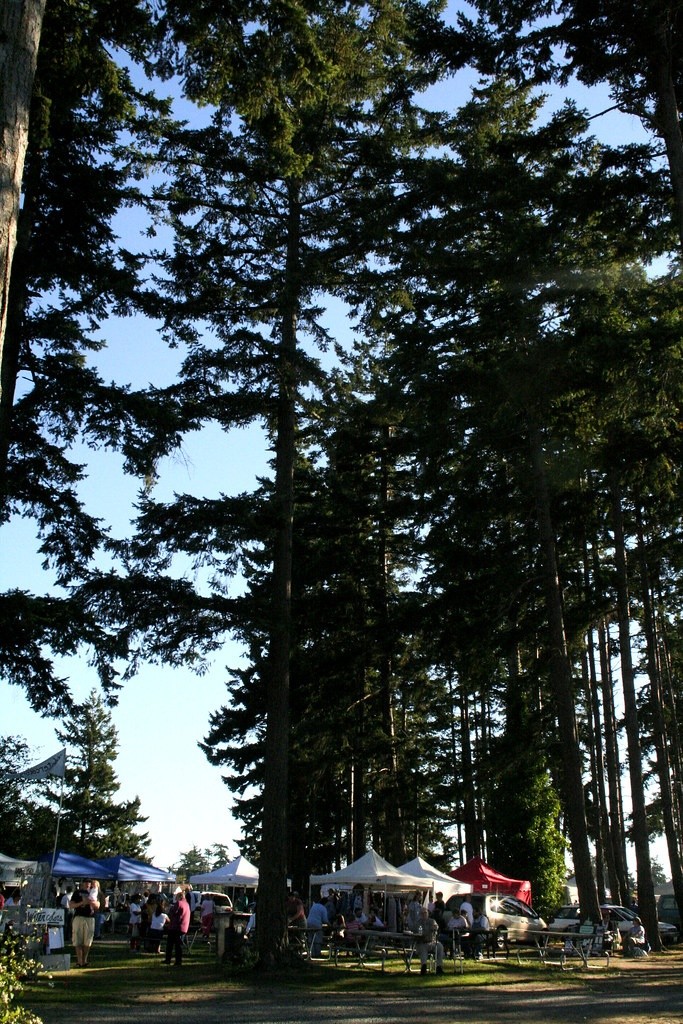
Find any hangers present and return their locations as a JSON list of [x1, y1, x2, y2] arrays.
[[389, 893, 410, 899]]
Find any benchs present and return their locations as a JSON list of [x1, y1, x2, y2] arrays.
[[376, 945, 435, 956], [548, 947, 605, 954], [514, 945, 574, 956], [306, 940, 334, 947], [333, 946, 401, 959]]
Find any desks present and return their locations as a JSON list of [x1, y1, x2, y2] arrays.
[[158, 925, 211, 956], [334, 929, 432, 974], [517, 930, 611, 970], [458, 928, 510, 957], [288, 927, 333, 962]]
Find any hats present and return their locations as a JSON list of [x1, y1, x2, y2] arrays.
[[66, 885, 73, 890], [11, 889, 22, 898], [634, 917, 643, 926], [83, 878, 92, 883]]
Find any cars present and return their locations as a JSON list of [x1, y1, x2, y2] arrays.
[[191, 891, 233, 928], [548, 903, 678, 944], [447, 892, 549, 950]]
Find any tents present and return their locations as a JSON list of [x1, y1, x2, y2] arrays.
[[0, 850, 534, 935]]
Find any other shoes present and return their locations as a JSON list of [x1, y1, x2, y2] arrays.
[[421, 969, 428, 975], [475, 954, 483, 960], [436, 966, 445, 974], [130, 948, 139, 952], [83, 963, 90, 967], [173, 963, 181, 967], [161, 959, 170, 964], [76, 964, 82, 967]]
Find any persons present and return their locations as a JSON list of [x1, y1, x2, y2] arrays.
[[0, 878, 645, 977]]
